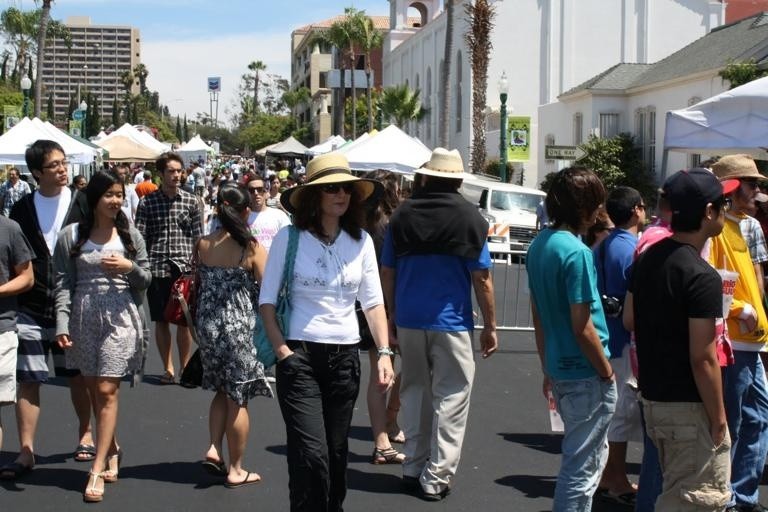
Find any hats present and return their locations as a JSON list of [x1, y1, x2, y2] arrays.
[[709, 153, 768, 186], [660, 166, 741, 221], [277, 156, 385, 218], [753, 183, 768, 204], [414, 146, 467, 180]]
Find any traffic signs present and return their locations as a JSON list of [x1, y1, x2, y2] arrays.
[[545, 147, 587, 161]]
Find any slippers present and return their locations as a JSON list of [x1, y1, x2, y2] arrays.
[[1, 460, 33, 479], [601, 489, 641, 507], [73, 443, 97, 463], [160, 373, 175, 385], [201, 458, 263, 490]]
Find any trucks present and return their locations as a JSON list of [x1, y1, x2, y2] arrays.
[[460, 174, 550, 257]]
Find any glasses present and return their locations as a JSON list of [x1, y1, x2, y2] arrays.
[[318, 181, 354, 195], [715, 197, 734, 212], [742, 177, 759, 190], [630, 203, 648, 210], [247, 186, 264, 194]]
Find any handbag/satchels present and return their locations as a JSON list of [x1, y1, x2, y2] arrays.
[[160, 274, 197, 329], [255, 291, 288, 367]]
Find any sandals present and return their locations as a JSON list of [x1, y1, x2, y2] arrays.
[[83, 445, 122, 503], [370, 445, 408, 465], [384, 422, 409, 445]]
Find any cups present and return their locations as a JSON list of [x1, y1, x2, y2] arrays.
[[711, 268, 739, 320], [547, 392, 564, 431]]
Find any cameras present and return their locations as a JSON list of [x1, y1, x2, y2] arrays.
[[600, 295, 624, 319]]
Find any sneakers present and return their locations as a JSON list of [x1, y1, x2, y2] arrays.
[[421, 473, 457, 502]]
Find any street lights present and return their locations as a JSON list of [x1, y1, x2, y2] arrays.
[[21, 74, 32, 120], [160, 97, 185, 122], [80, 100, 88, 139], [498, 72, 509, 183]]
